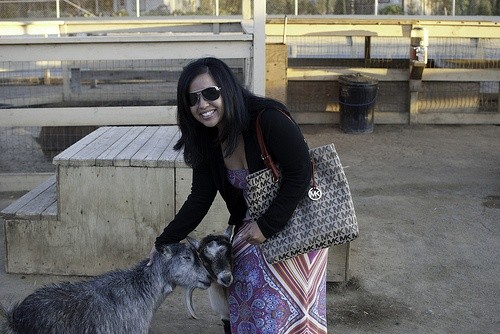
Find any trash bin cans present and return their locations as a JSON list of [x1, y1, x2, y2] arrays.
[[338, 73, 377, 134]]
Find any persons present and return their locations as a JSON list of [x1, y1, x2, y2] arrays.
[[146, 58, 329, 334]]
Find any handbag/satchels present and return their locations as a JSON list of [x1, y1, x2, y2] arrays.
[[244, 106, 360, 264]]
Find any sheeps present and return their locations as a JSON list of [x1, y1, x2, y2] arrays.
[[17, 225, 236, 334]]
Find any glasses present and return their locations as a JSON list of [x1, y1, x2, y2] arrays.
[[183, 86, 221, 107]]
